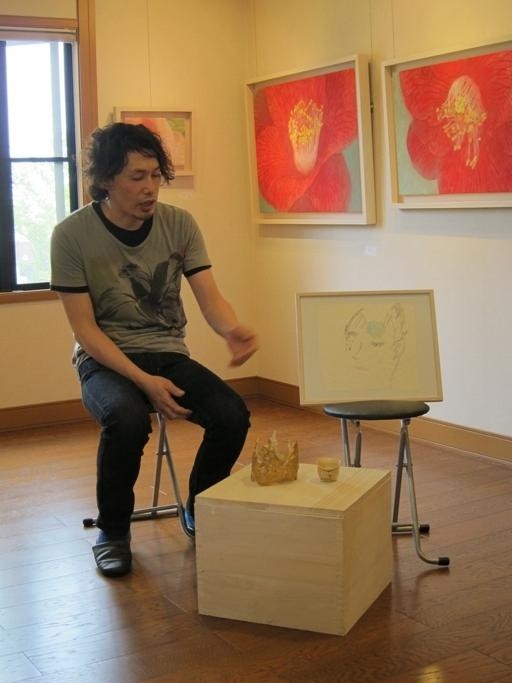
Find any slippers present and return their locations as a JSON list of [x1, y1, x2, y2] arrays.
[[92, 538, 131, 578]]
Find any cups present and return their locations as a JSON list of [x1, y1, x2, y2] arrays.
[[318, 456, 341, 481]]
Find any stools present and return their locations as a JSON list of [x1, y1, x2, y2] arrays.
[[194, 460, 393, 637], [323, 401, 451, 565], [82, 397, 195, 540]]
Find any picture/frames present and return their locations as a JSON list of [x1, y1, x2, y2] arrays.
[[112, 105, 194, 177], [295, 288, 444, 406], [380, 37, 512, 209], [244, 55, 376, 225]]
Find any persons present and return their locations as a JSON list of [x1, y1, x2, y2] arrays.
[[49, 122, 262, 577]]
